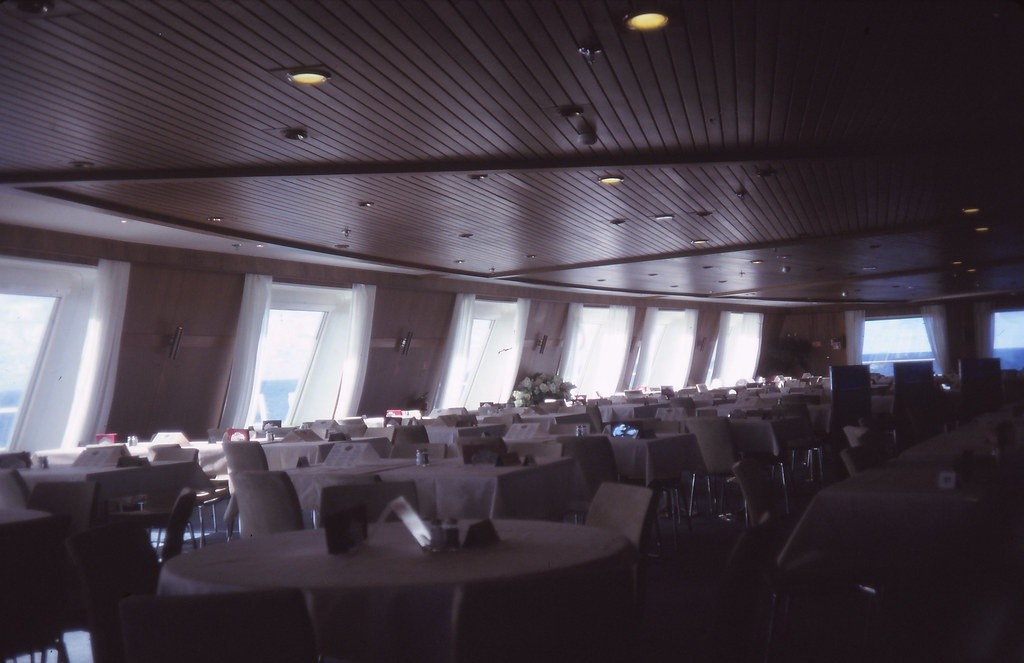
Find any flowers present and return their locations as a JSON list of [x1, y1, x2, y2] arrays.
[[510, 373, 578, 408]]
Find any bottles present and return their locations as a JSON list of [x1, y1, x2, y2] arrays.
[[416, 448, 430, 468], [127, 436, 137, 446], [576, 425, 587, 437], [37, 456, 47, 468], [429, 516, 460, 552]]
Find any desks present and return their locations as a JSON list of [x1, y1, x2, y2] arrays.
[[11, 460, 216, 526], [766, 399, 1023, 663], [655, 417, 811, 518], [598, 403, 669, 424], [279, 458, 580, 519], [364, 422, 505, 447], [477, 413, 598, 435], [37, 441, 228, 479], [158, 519, 636, 663], [254, 437, 389, 472], [502, 434, 707, 555]]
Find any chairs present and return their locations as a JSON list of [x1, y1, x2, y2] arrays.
[[0, 357, 1024, 663]]
